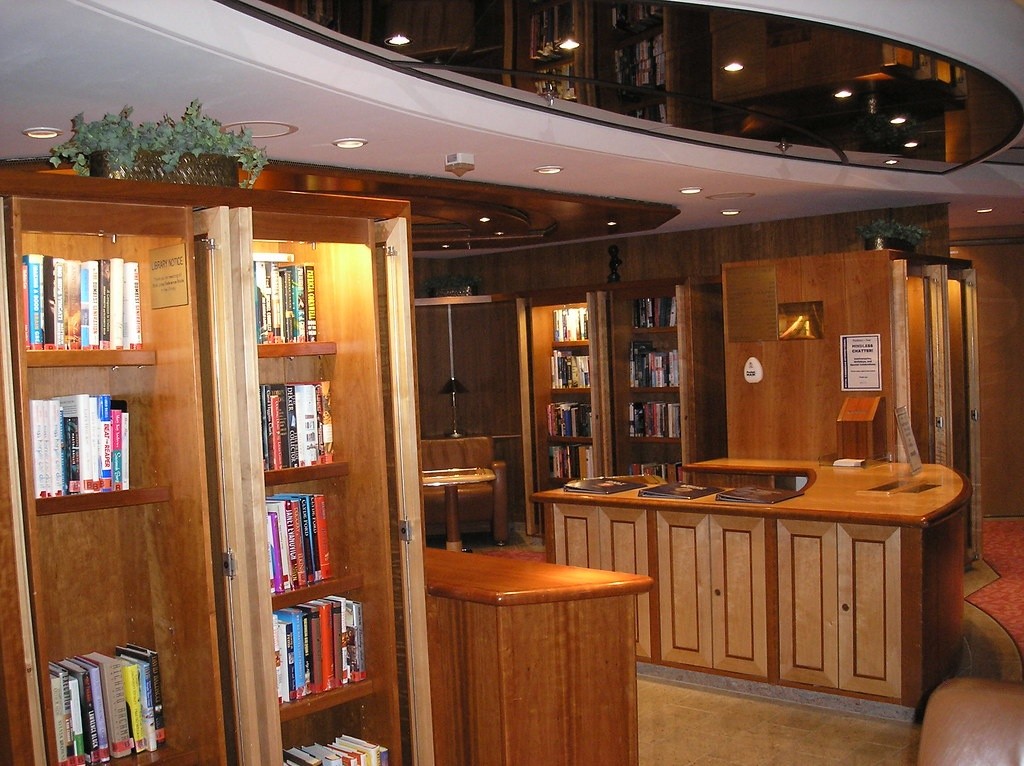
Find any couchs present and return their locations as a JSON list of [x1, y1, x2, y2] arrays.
[[421, 435, 514, 547]]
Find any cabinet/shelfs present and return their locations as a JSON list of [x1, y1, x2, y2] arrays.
[[515, 0, 596, 107], [528, 283, 713, 539], [594, 0, 715, 133], [0, 167, 441, 766]]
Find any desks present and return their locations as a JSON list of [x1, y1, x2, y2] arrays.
[[422, 469, 497, 551], [424, 546, 655, 766]]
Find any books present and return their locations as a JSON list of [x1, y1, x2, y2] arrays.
[[252, 261, 365, 701], [27, 395, 132, 501], [19, 256, 142, 351], [286, 735, 386, 766], [47, 640, 165, 766], [529, 2, 667, 123], [546, 306, 595, 479], [626, 296, 682, 484]]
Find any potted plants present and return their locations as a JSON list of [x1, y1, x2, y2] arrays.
[[49, 95, 271, 188], [855, 217, 932, 252], [416, 259, 489, 298]]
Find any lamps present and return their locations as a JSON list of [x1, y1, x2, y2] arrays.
[[438, 378, 469, 438]]
